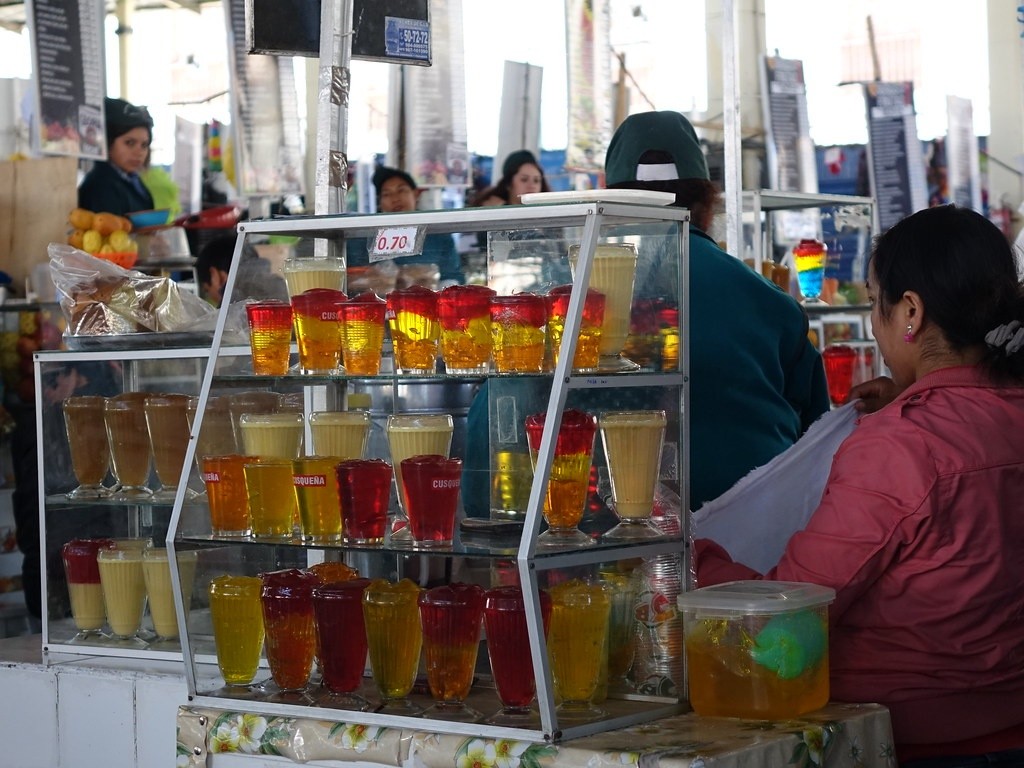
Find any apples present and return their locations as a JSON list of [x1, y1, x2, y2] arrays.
[[16, 310, 69, 375]]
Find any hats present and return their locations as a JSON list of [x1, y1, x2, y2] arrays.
[[105, 96, 150, 145], [604, 110, 710, 186]]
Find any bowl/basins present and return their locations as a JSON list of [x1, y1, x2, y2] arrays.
[[127, 210, 172, 225]]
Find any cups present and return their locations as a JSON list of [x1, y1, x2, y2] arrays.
[[822, 353, 855, 407], [740, 246, 830, 306], [489, 298, 549, 374], [384, 292, 440, 376], [439, 290, 496, 373], [546, 294, 605, 371], [286, 253, 348, 374], [291, 300, 343, 377], [64, 385, 700, 724], [569, 243, 643, 373], [247, 303, 298, 377], [333, 301, 392, 378]]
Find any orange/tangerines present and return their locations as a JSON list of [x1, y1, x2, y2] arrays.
[[68, 208, 132, 250]]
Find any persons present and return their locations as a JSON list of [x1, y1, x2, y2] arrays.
[[691, 200, 1024, 768], [341, 150, 575, 298], [463, 110, 831, 537], [13, 99, 295, 639]]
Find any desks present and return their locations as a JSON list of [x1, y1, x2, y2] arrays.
[[177, 669, 894, 768]]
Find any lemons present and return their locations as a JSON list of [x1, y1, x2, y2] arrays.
[[83, 230, 138, 254], [0, 310, 38, 370]]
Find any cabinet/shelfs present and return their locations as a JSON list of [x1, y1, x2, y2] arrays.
[[34, 185, 874, 743]]
